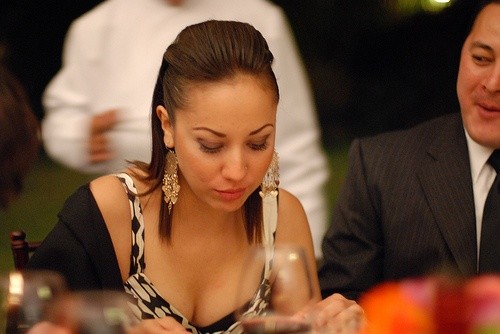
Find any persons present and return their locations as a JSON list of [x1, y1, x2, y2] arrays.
[[318, 0, 499, 306], [39, 1, 332, 262], [24, 18, 368, 334]]
